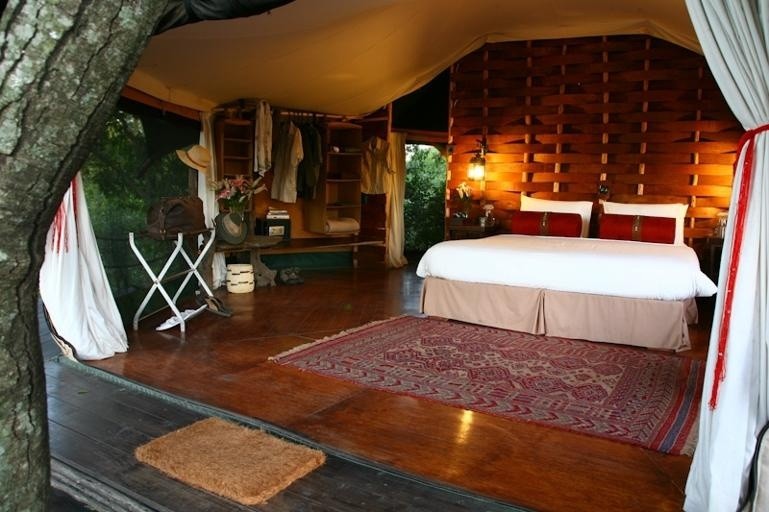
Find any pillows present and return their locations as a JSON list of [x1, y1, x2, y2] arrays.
[[511, 193, 689, 246]]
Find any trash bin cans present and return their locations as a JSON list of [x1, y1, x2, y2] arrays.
[[227, 264, 254, 293]]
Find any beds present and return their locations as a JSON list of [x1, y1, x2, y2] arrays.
[[420, 191, 692, 353]]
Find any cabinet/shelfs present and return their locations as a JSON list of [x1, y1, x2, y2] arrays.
[[214, 108, 390, 278]]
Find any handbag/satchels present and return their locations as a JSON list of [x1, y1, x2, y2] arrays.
[[148, 196, 206, 232]]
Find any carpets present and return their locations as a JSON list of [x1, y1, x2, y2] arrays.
[[133, 417, 327, 507], [270, 314, 708, 459]]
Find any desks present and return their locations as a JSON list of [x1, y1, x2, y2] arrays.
[[128, 228, 217, 336]]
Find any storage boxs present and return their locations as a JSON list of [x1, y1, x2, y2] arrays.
[[257, 217, 291, 243]]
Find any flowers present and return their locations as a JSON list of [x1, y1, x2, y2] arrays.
[[207, 174, 268, 212]]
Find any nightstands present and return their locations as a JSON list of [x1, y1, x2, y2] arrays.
[[700, 237, 725, 326], [447, 224, 498, 240]]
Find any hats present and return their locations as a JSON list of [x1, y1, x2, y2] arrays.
[[216, 212, 248, 244], [176, 145, 211, 171]]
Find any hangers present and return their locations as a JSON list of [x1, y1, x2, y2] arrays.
[[286, 110, 317, 127]]
